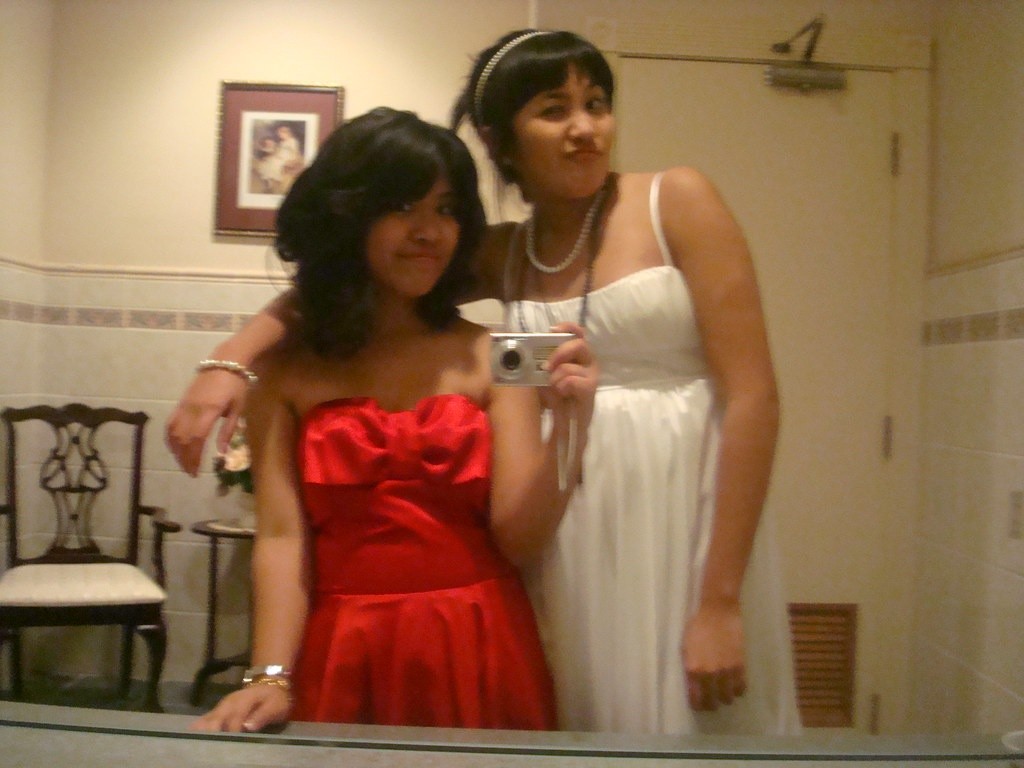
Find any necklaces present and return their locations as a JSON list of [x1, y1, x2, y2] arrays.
[[527, 197, 598, 273]]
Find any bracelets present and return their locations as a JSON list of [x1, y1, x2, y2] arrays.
[[244, 664, 293, 690], [195, 360, 258, 387]]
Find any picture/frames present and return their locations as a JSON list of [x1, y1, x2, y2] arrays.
[[214, 80, 345, 238]]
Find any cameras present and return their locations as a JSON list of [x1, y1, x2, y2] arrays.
[[488, 332, 578, 387]]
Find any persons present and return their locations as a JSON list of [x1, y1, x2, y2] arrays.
[[188, 105, 601, 734], [256, 125, 298, 194], [167, 28, 800, 737]]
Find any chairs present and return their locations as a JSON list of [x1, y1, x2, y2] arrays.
[[0, 400, 183, 712]]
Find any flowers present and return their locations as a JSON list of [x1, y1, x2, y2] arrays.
[[209, 416, 253, 497]]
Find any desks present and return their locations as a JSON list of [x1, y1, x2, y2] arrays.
[[189, 518, 255, 705]]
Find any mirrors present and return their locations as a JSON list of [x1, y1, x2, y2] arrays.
[[0, 0, 1024, 760]]
